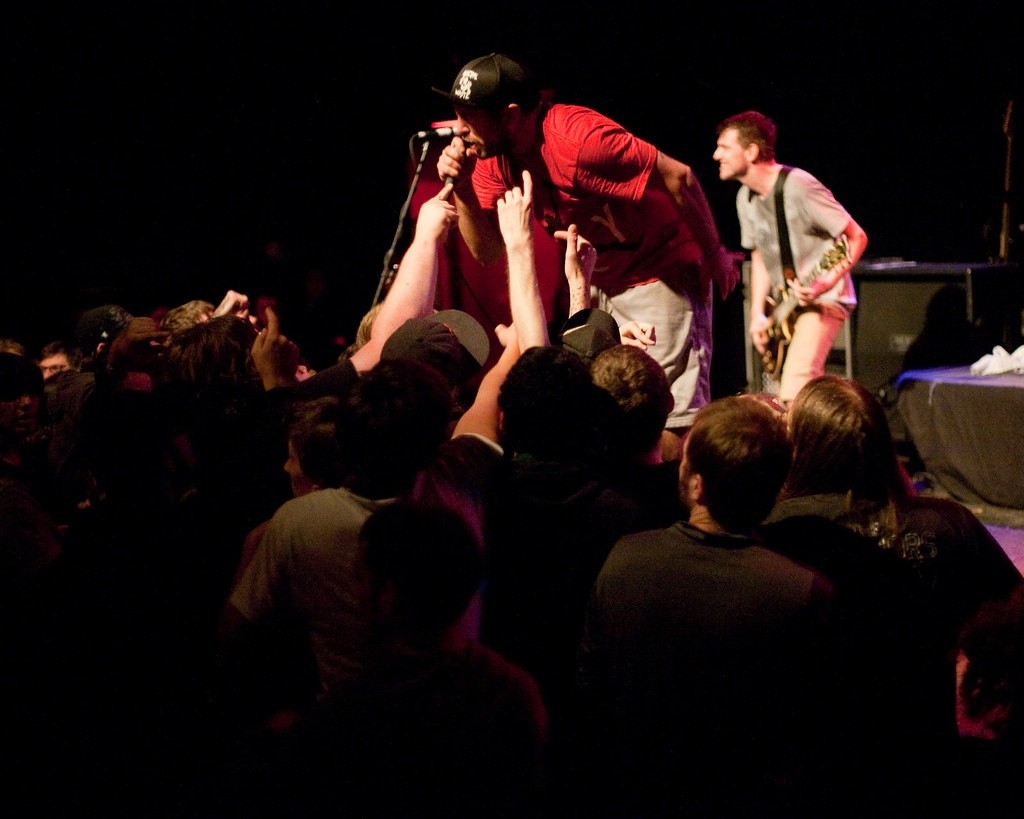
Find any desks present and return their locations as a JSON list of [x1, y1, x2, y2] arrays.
[[894, 364, 1024, 529]]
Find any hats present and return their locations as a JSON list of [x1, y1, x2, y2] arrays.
[[381, 308, 490, 415], [431, 52, 540, 111], [558, 308, 623, 366]]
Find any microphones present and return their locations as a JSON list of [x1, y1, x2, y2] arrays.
[[444, 140, 472, 201], [417, 123, 460, 140]]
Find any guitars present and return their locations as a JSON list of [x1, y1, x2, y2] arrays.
[[755, 233, 850, 380]]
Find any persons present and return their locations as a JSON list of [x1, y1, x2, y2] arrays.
[[0, 51, 1024, 818]]
